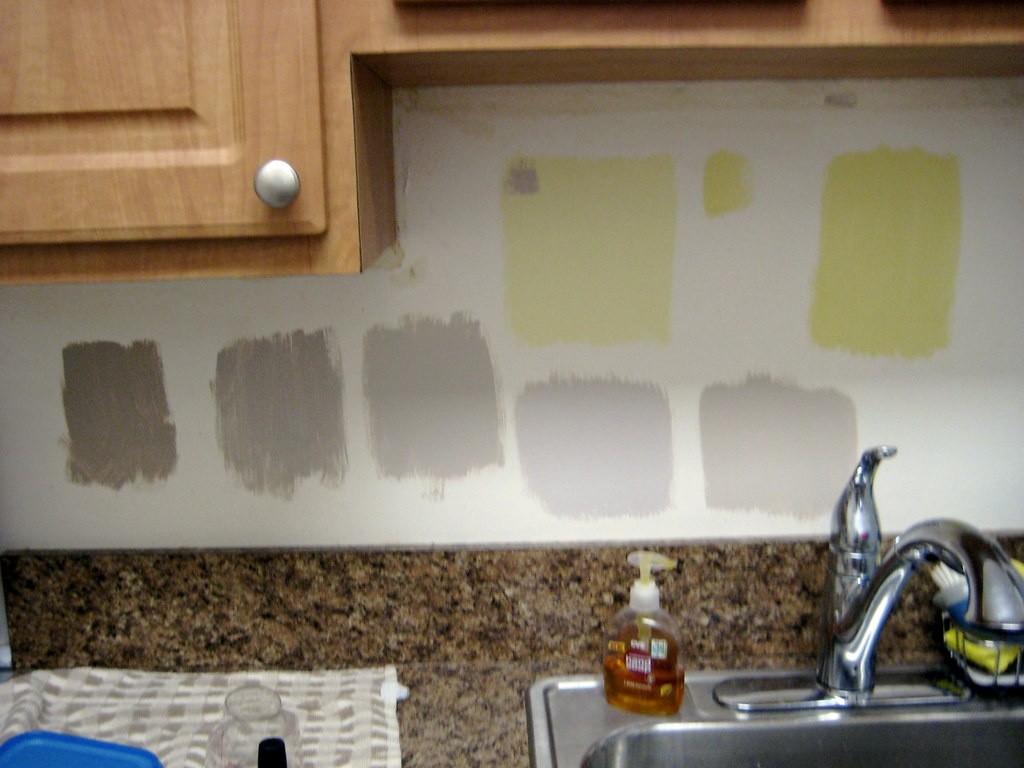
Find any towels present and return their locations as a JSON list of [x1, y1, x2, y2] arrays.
[[2, 665, 410, 766]]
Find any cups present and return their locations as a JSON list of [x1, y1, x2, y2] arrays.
[[221, 686, 304, 768]]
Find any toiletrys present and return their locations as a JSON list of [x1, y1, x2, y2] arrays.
[[603, 548, 686, 717]]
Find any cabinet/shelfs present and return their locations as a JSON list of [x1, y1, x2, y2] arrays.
[[0, 0, 1024, 283]]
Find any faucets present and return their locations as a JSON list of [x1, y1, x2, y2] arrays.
[[812, 445, 1023, 693]]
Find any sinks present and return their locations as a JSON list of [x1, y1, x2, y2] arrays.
[[527, 665, 1022, 766]]
